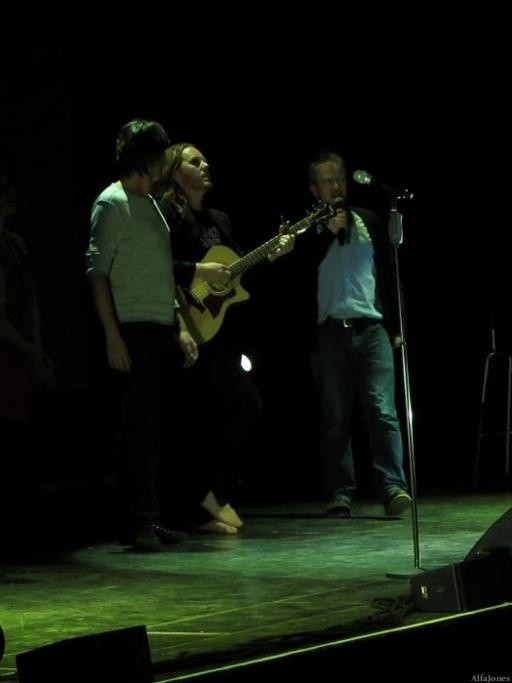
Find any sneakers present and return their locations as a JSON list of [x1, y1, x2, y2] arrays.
[[130, 510, 188, 553], [325, 498, 354, 518], [196, 490, 245, 535], [383, 486, 413, 516]]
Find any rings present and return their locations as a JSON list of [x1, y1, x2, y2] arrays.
[[332, 217, 335, 222]]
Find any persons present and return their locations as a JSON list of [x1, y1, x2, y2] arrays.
[[291, 150, 413, 518], [155, 141, 296, 536], [86, 119, 200, 548]]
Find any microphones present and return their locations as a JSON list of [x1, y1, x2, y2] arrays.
[[353, 170, 414, 202], [333, 196, 350, 246]]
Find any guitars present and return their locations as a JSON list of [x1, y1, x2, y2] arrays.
[[182, 199, 346, 345]]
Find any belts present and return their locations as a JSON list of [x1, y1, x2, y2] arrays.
[[328, 317, 362, 330]]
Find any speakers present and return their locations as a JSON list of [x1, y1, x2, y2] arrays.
[[461, 507, 512, 610], [15, 624, 156, 682]]
